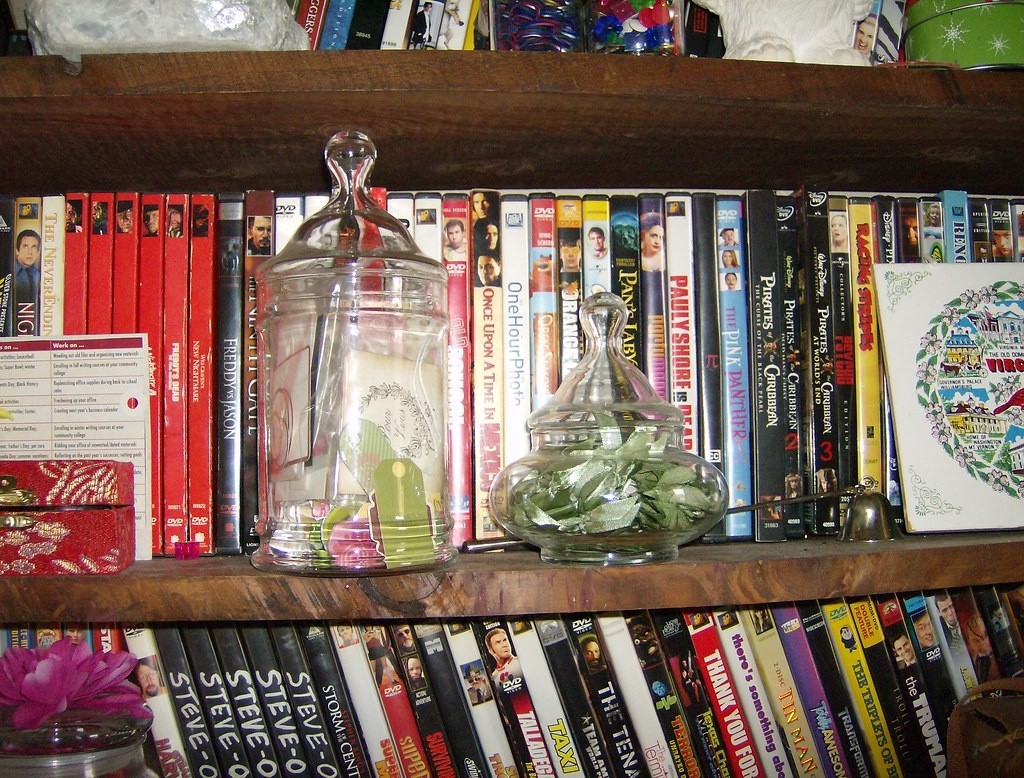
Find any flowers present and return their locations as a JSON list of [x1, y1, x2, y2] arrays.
[[0, 636, 156, 729]]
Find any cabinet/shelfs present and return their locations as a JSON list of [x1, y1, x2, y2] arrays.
[[0, 0, 1024, 778]]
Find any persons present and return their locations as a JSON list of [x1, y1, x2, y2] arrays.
[[463, 664, 492, 706], [588, 227, 606, 258], [640, 213, 664, 266], [65, 622, 86, 646], [912, 611, 937, 650], [485, 628, 524, 695], [443, 218, 468, 264], [5, 229, 41, 336], [934, 589, 966, 656], [336, 621, 360, 648], [134, 658, 166, 701], [893, 632, 917, 671], [393, 653, 427, 691], [248, 216, 271, 256], [579, 636, 607, 677], [393, 624, 417, 652]]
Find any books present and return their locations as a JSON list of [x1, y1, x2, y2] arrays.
[[0, 586, 1024, 778], [283, 0, 495, 50], [0, 186, 1024, 560], [684, 0, 726, 58]]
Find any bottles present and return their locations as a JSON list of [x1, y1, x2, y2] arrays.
[[251, 131, 459, 575], [489, 292, 730, 567]]
